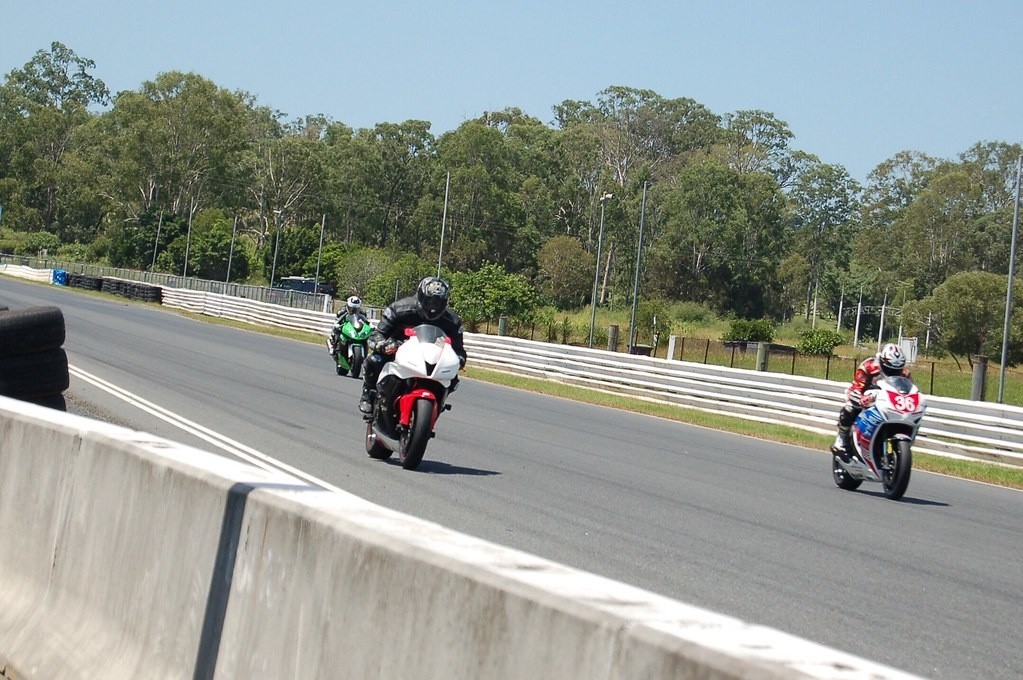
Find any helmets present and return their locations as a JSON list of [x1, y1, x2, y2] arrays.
[[417, 277, 448, 321], [878, 343, 906, 380], [346, 296, 360, 315]]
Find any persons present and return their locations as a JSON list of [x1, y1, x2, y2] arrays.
[[330, 296, 369, 358], [358, 276, 468, 413], [834, 345, 913, 458]]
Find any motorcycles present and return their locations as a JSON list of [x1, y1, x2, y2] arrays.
[[359, 323, 464, 469], [326, 312, 370, 377], [830, 374, 927, 499]]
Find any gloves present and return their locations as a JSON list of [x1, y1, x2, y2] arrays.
[[379, 337, 397, 354], [860, 394, 876, 409]]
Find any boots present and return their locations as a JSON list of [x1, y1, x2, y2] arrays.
[[833, 423, 851, 455], [358, 355, 382, 414]]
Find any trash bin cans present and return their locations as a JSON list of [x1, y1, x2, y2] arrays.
[[608, 325, 619, 351], [755, 342, 769, 371], [499, 316, 507, 336], [627, 344, 654, 356]]
